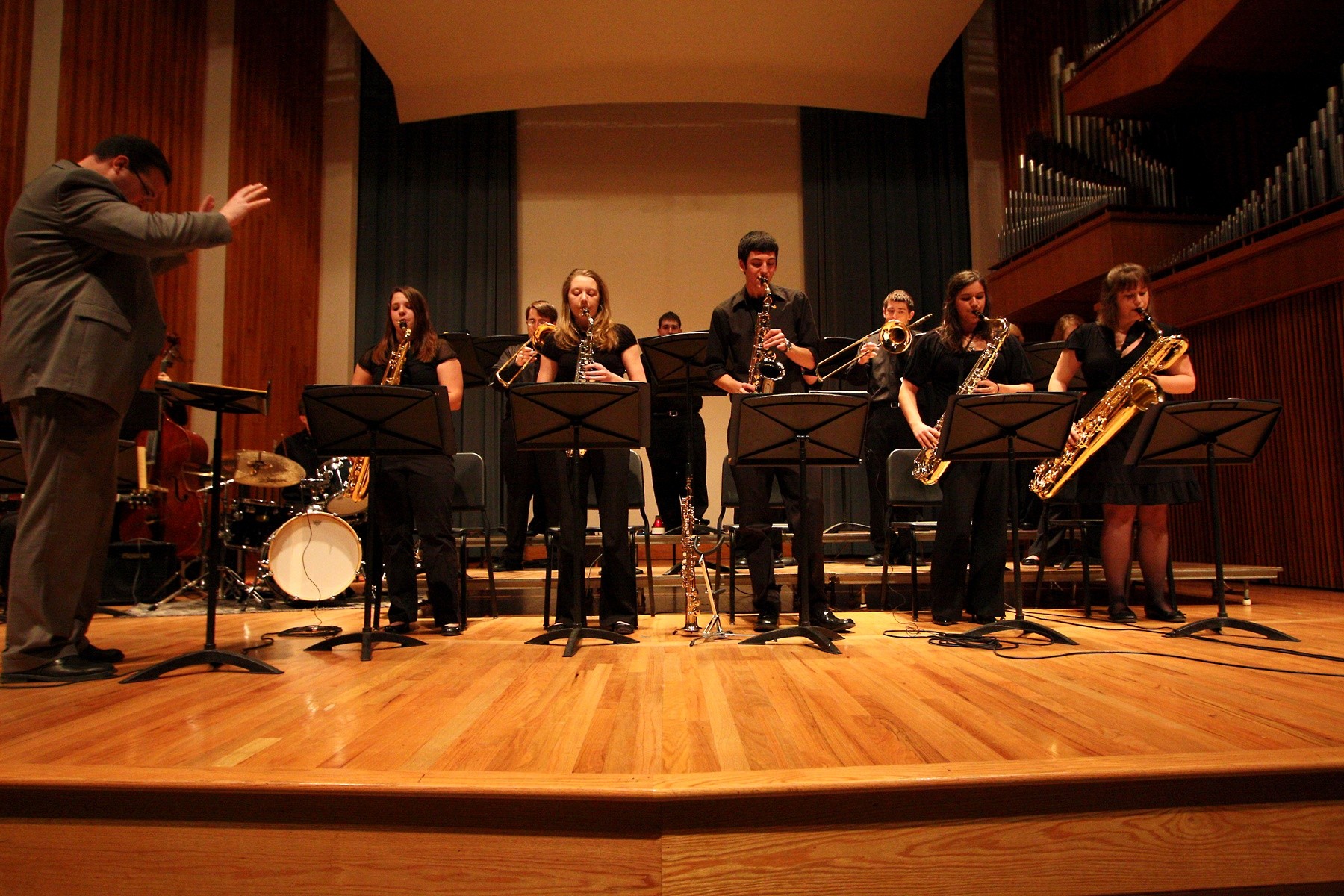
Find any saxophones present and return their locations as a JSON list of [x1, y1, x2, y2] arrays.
[[910, 310, 1012, 486], [1027, 306, 1190, 501], [749, 275, 785, 407], [679, 462, 704, 632], [564, 309, 598, 458], [345, 318, 413, 501]]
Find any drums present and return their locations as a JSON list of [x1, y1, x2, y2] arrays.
[[316, 455, 370, 518], [223, 496, 300, 551], [298, 476, 328, 514], [257, 505, 364, 605]]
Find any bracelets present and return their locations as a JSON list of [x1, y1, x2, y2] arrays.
[[784, 336, 792, 353]]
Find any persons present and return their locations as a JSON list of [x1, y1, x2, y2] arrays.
[[491, 299, 559, 571], [904, 276, 1034, 626], [708, 231, 856, 632], [645, 310, 711, 536], [845, 290, 926, 566], [1048, 263, 1196, 623], [351, 285, 464, 635], [1, 133, 271, 679], [1000, 312, 1084, 566], [538, 269, 647, 634]]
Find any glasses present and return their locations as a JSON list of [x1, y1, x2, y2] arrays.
[[526, 320, 550, 327], [128, 163, 157, 199]]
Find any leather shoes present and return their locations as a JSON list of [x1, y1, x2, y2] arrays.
[[61, 643, 125, 668], [972, 611, 996, 626], [865, 553, 885, 565], [800, 608, 855, 632], [934, 613, 953, 626], [1143, 598, 1186, 621], [897, 551, 926, 566], [1108, 602, 1136, 621], [754, 609, 780, 630], [0, 658, 117, 683], [735, 556, 751, 568], [773, 558, 783, 567]]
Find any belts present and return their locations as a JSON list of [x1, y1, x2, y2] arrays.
[[656, 410, 685, 417]]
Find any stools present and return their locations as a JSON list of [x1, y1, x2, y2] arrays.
[[102, 540, 177, 609]]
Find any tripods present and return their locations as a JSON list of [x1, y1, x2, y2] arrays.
[[1121, 398, 1303, 642], [935, 340, 1106, 645], [119, 381, 284, 684], [302, 384, 456, 662], [508, 381, 651, 658], [640, 328, 874, 650]]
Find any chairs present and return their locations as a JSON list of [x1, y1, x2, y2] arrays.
[[878, 448, 944, 622], [713, 455, 794, 628], [413, 452, 498, 632], [543, 450, 655, 629], [1035, 479, 1177, 617]]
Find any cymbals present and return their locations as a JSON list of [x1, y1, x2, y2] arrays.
[[209, 450, 307, 489], [183, 469, 227, 480]]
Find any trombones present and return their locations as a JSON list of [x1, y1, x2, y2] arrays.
[[815, 311, 935, 383], [496, 323, 560, 389]]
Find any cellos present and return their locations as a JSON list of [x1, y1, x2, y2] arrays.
[[118, 330, 209, 559]]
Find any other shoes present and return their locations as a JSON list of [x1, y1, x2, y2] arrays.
[[616, 620, 635, 633], [492, 562, 523, 572], [1021, 556, 1040, 565], [441, 620, 462, 635], [548, 619, 587, 631], [384, 621, 410, 633]]
